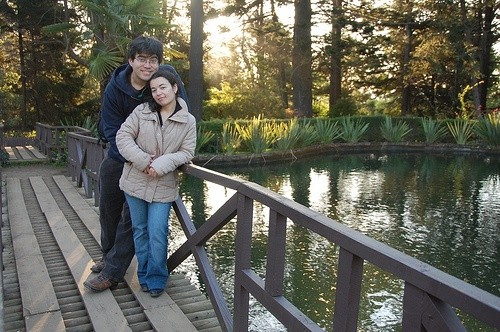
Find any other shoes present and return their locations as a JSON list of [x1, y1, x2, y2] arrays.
[[85, 272, 118, 292], [90, 261, 107, 272]]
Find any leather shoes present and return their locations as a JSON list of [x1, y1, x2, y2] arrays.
[[141, 283, 149, 291], [149, 288, 164, 296]]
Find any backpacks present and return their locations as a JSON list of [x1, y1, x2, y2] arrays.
[[95, 72, 114, 146]]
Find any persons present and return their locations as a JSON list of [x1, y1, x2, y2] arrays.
[[83, 36, 190, 292], [116, 70, 196, 298]]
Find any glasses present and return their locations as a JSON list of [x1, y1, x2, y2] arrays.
[[134, 57, 158, 65]]
